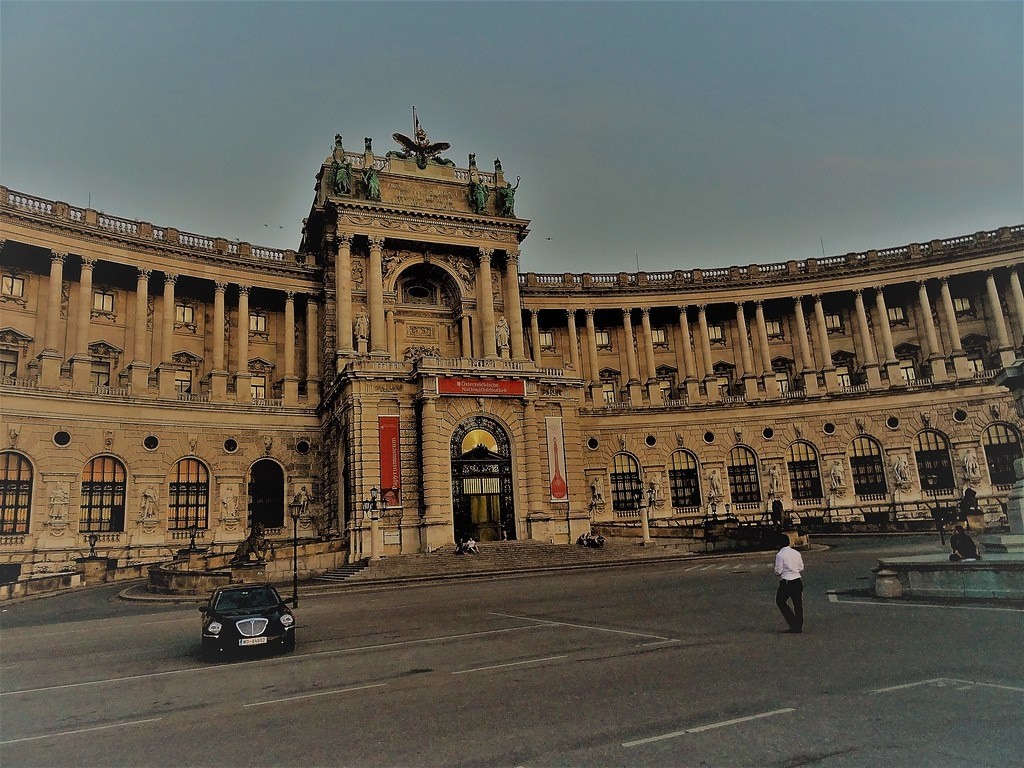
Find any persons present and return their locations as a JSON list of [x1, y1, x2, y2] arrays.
[[496, 316, 510, 348], [355, 306, 369, 337], [830, 460, 846, 490], [221, 485, 238, 518], [949, 525, 982, 561], [382, 249, 407, 281], [963, 448, 981, 478], [448, 253, 471, 279], [50, 482, 71, 521], [709, 469, 721, 496], [645, 480, 658, 519], [768, 463, 784, 492], [590, 476, 604, 501], [468, 175, 521, 214], [893, 454, 911, 481], [294, 487, 314, 514], [142, 484, 161, 518], [332, 155, 387, 198], [455, 538, 481, 555], [776, 535, 804, 631], [576, 532, 606, 548], [402, 345, 442, 363]]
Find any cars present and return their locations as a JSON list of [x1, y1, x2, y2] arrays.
[[199, 581, 297, 660]]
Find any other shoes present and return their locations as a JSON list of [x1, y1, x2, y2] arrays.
[[779, 627, 803, 633]]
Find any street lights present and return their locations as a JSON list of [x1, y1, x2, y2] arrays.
[[287, 495, 303, 612]]
